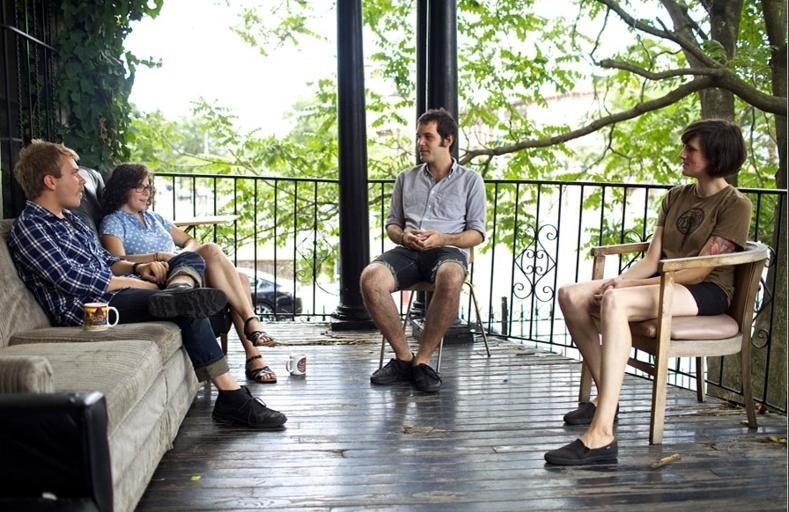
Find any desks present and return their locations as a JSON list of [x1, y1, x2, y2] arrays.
[[171, 213, 241, 243]]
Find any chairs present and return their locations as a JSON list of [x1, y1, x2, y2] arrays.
[[577, 238, 767, 445], [377, 246, 492, 376], [70, 163, 233, 355]]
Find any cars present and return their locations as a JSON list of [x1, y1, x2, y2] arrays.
[[230, 266, 306, 327], [151, 173, 216, 212]]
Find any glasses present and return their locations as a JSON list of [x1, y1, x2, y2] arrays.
[[132, 184, 153, 193]]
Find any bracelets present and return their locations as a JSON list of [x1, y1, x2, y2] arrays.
[[153, 252, 160, 263], [131, 261, 143, 277]]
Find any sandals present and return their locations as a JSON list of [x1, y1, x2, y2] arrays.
[[244, 355, 278, 383], [242, 316, 274, 346]]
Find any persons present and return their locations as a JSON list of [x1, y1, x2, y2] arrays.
[[360, 108, 486, 390], [543, 118, 752, 467], [99, 162, 276, 384], [7, 139, 288, 428]]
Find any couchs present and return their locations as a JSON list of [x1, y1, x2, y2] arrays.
[[0, 217, 208, 512]]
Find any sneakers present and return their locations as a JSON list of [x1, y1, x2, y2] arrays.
[[370, 352, 415, 386], [147, 283, 228, 320], [211, 386, 287, 428], [543, 437, 619, 465], [563, 400, 620, 425], [411, 363, 443, 393]]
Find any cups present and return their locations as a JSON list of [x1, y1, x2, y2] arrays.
[[84, 303, 119, 332], [287, 352, 307, 376]]
